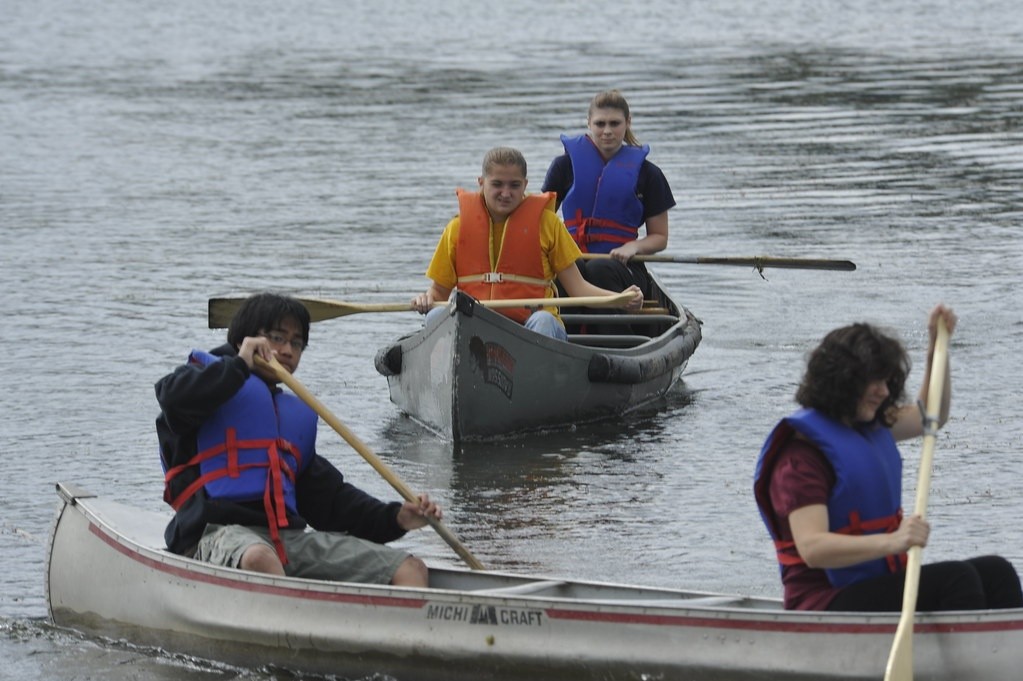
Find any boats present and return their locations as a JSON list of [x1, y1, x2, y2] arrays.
[[374, 265, 702, 445], [45, 480, 1023, 681]]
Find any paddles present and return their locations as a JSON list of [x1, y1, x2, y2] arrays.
[[255, 346, 486, 569], [580, 252, 857, 272], [879, 315, 950, 680], [208, 291, 634, 331]]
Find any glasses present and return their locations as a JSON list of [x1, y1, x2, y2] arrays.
[[258, 332, 307, 350]]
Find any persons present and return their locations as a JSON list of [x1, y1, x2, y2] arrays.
[[411, 146, 644, 342], [154, 293, 442, 588], [541, 91, 676, 348], [753, 304, 1023, 613]]
